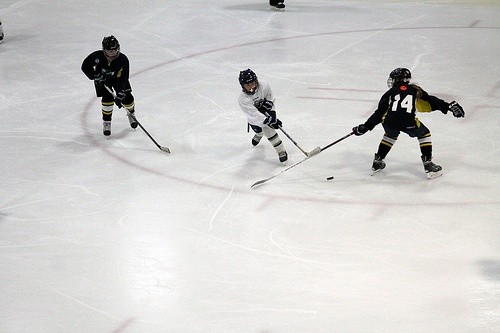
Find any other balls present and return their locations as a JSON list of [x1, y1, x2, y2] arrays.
[[327, 176, 334, 181]]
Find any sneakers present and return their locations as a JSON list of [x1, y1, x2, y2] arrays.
[[425, 163, 443, 179], [102, 118, 112, 140], [279, 151, 288, 166], [128, 112, 139, 132], [0, 22, 5, 44], [269, 1, 285, 11], [370, 162, 387, 176], [252, 134, 264, 148]]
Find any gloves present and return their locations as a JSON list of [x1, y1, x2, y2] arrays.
[[259, 100, 273, 114], [94, 73, 106, 86], [353, 124, 367, 136], [113, 92, 125, 108], [263, 117, 283, 130], [448, 101, 465, 118]]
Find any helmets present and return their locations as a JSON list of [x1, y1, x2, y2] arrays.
[[387, 69, 411, 88], [102, 36, 120, 59], [239, 69, 258, 95]]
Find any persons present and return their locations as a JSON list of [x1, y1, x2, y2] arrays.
[[0, 22, 4, 44], [238, 68, 288, 166], [353, 68, 465, 179], [81, 35, 138, 139], [269, 0, 285, 12]]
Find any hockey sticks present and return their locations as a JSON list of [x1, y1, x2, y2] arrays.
[[251, 131, 354, 188], [103, 84, 170, 154], [265, 111, 321, 158]]
